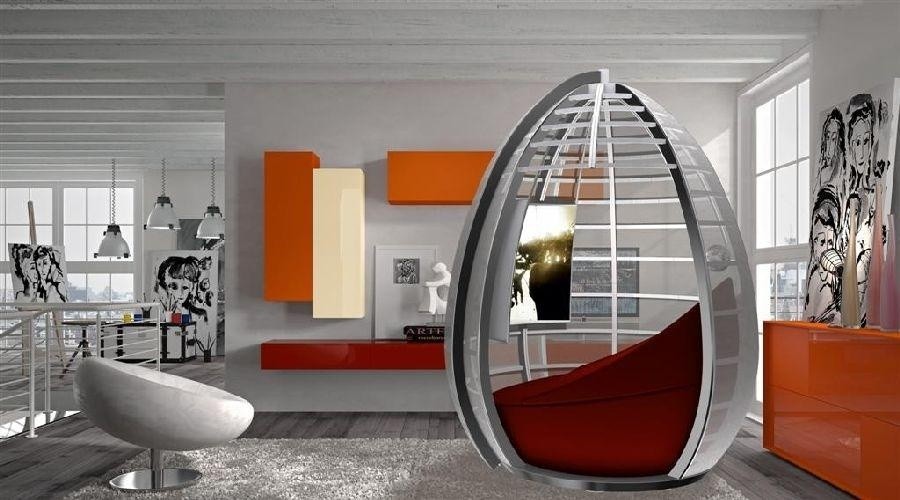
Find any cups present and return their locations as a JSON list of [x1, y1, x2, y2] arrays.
[[172, 313, 181, 324], [123, 314, 131, 323], [143, 311, 150, 318], [166, 315, 172, 323], [134, 314, 142, 322], [182, 314, 189, 324]]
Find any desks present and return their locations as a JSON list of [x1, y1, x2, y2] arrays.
[[762, 320, 900, 498], [60, 320, 197, 379]]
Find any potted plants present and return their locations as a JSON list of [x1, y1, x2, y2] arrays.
[[196, 331, 216, 362]]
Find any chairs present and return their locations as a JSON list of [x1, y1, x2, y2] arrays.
[[74, 357, 254, 493], [493, 279, 735, 477]]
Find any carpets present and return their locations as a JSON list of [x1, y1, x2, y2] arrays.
[[64, 435, 740, 498]]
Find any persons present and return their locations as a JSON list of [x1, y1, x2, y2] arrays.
[[807, 186, 844, 325], [812, 107, 845, 191], [844, 94, 889, 191], [10, 244, 44, 302], [34, 246, 66, 302], [155, 254, 208, 321]]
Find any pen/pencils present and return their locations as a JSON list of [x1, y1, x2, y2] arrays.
[[136, 293, 155, 310], [160, 292, 177, 310]]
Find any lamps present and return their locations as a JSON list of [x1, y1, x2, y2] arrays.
[[93, 157, 226, 259]]
